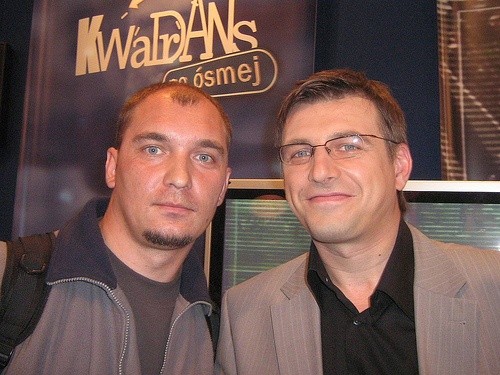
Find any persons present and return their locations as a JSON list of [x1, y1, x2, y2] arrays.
[[217, 72, 500, 375], [0, 82, 232, 375]]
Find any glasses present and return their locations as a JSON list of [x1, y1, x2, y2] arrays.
[[276, 134, 369, 166]]
